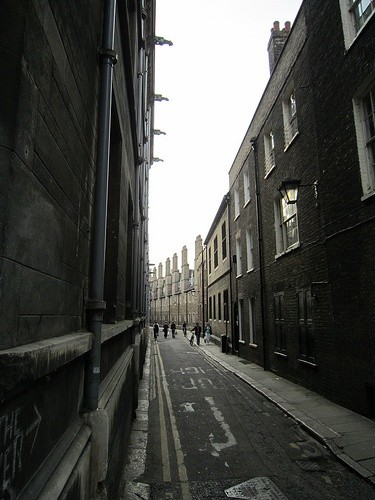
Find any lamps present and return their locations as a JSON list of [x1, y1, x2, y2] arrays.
[[276, 178, 319, 207]]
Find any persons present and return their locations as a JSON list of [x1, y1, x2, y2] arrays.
[[204, 323, 212, 344], [171, 322, 176, 338], [182, 321, 187, 336], [163, 321, 169, 338], [153, 321, 159, 338], [190, 323, 201, 346]]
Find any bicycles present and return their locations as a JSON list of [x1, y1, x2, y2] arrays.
[[188, 332, 195, 346]]
